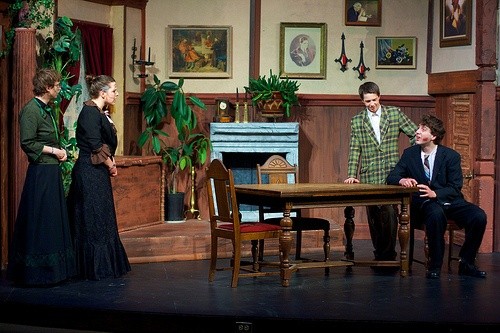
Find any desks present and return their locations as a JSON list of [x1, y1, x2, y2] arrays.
[[222, 185, 418, 287]]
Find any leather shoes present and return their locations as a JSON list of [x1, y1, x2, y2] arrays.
[[458, 261, 487, 276], [426, 270, 441, 279]]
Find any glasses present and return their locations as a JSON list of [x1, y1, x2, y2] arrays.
[[54, 84, 60, 88]]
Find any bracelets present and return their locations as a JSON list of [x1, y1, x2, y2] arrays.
[[52, 147, 54, 155]]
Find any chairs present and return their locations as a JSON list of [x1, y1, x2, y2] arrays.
[[251, 155, 333, 280], [406, 195, 466, 280], [203, 158, 285, 288]]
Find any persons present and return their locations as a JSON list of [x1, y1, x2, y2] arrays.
[[6, 68, 81, 287], [345, 82, 419, 261], [66, 75, 131, 282], [385, 114, 487, 279]]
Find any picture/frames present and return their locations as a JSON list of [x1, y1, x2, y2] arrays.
[[279, 22, 327, 80], [375, 36, 417, 69], [167, 24, 232, 78], [345, 0, 383, 27], [438, 0, 472, 47]]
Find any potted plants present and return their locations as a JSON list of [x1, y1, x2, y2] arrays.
[[245, 70, 300, 116], [135, 75, 212, 222]]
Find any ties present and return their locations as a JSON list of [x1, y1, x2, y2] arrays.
[[424, 155, 430, 184]]
